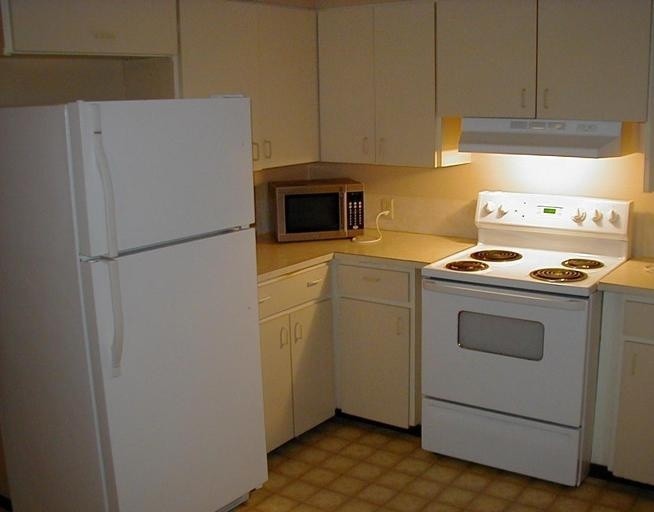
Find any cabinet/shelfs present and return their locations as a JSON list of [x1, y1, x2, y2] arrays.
[[334, 262, 413, 428], [613, 299, 654, 488], [435, 0, 649, 119], [9, 0, 174, 55], [256, 260, 335, 454], [317, 1, 435, 168], [178, 1, 320, 170]]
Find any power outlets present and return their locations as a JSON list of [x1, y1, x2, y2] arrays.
[[381, 198, 394, 220]]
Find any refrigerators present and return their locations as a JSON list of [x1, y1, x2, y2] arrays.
[[0, 98, 267, 511]]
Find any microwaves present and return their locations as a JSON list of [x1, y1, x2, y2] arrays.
[[269, 178, 366, 243]]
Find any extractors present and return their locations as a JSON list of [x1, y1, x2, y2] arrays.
[[459, 119, 644, 158]]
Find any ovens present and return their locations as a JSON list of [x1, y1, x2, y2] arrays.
[[421, 277, 603, 428]]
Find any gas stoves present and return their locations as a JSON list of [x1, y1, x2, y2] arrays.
[[422, 190, 633, 297]]
